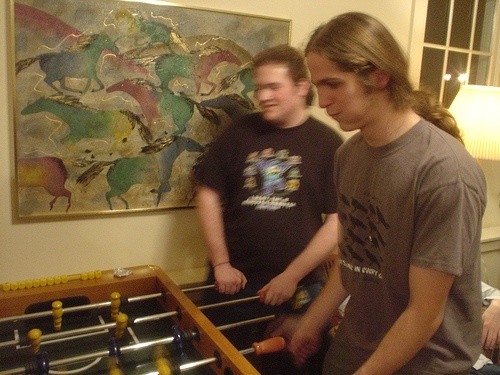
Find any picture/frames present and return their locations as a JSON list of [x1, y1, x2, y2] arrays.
[[5, 0, 295, 227]]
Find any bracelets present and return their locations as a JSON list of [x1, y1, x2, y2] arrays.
[[212, 261, 231, 267]]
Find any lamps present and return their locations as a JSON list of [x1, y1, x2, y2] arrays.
[[443, 83, 500, 163]]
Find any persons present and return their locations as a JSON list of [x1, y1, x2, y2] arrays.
[[192, 45, 344, 375], [289, 11, 488, 375]]
